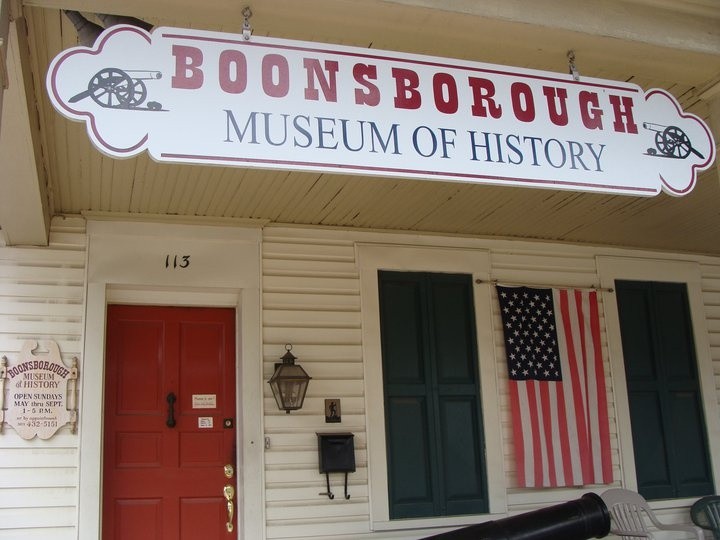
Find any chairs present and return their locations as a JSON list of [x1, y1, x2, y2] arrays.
[[690, 495, 720, 540], [599, 489, 706, 540]]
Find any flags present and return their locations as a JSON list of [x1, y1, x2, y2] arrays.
[[496, 281, 614, 488]]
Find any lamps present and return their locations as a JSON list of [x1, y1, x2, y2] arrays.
[[266, 343, 313, 414]]
